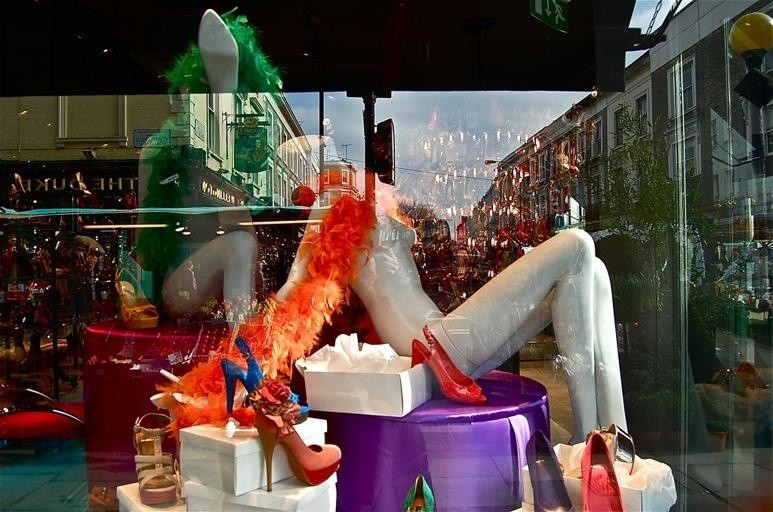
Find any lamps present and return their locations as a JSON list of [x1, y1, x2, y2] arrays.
[[82, 149, 96, 161]]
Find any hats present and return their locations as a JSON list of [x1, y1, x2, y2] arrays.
[[72, 235, 106, 257]]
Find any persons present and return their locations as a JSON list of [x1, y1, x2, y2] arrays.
[[135, 9, 282, 331], [0, 228, 107, 368], [267, 133, 630, 443], [641, 219, 729, 497]]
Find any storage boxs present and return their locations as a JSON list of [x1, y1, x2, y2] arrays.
[[179, 468, 339, 512], [302, 354, 436, 419], [178, 413, 330, 498], [114, 481, 189, 512]]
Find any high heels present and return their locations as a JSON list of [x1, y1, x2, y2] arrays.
[[221, 338, 310, 424], [254, 410, 341, 492], [581, 432, 624, 511], [113, 268, 159, 329], [132, 412, 177, 506], [411, 326, 487, 405], [527, 429, 574, 512], [403, 474, 435, 512]]
